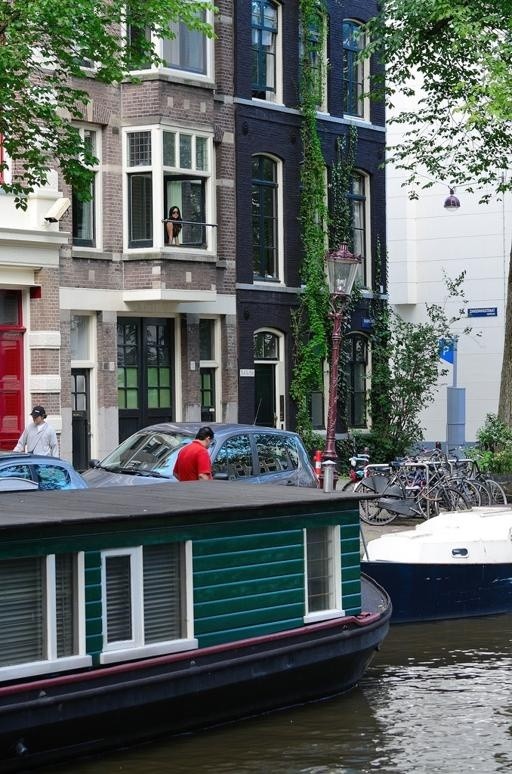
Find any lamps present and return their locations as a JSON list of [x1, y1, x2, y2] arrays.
[[442, 182, 461, 211]]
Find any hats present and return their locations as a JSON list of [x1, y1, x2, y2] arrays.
[[30, 407, 44, 416]]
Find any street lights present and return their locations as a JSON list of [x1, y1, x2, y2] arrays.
[[323, 236, 362, 495]]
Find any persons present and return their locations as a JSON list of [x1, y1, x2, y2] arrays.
[[173, 426, 214, 482], [13, 405, 60, 459], [164, 205, 183, 245]]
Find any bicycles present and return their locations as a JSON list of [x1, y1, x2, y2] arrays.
[[341, 445, 507, 526]]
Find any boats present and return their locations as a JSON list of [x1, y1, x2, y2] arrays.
[[0, 477, 393, 770], [356, 501, 512, 623]]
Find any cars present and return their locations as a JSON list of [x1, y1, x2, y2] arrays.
[[0, 448, 90, 494], [82, 422, 319, 487]]
